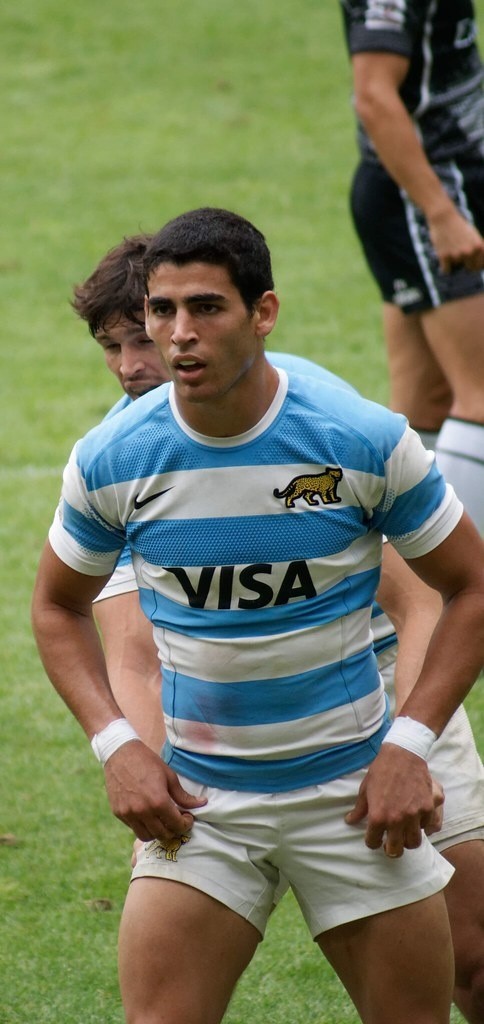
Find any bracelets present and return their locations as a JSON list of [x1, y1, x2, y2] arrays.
[[380, 716, 436, 762], [90, 718, 141, 766]]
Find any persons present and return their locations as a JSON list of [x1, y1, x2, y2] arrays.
[[339, 0, 484, 541], [32, 208, 484, 1024]]
